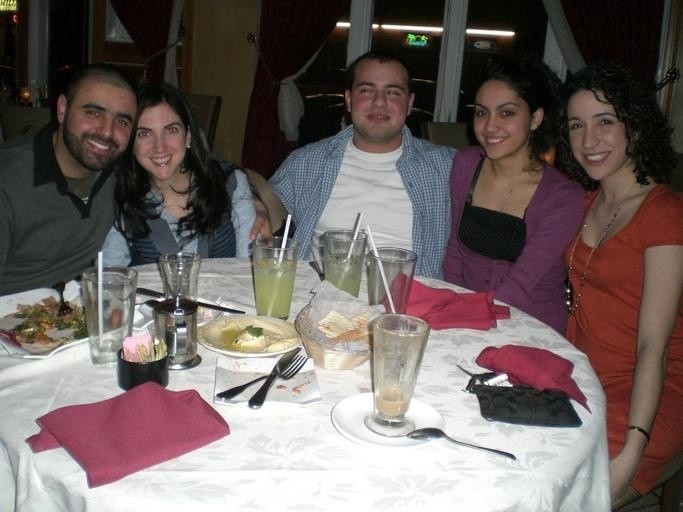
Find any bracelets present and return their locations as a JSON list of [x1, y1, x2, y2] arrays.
[[626, 423, 652, 446]]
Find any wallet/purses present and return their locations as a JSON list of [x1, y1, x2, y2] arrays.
[[473, 385, 583, 427]]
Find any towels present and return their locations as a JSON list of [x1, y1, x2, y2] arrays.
[[476, 345, 592, 414], [28, 381, 230, 487], [381, 273, 510, 330]]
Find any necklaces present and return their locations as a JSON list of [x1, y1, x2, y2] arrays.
[[564, 183, 638, 316]]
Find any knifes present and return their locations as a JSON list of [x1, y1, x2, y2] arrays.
[[247, 345, 298, 409], [128, 284, 247, 320]]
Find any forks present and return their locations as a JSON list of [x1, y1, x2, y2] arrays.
[[50, 281, 74, 318], [215, 355, 310, 401]]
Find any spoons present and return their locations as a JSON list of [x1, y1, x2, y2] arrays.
[[407, 427, 519, 464]]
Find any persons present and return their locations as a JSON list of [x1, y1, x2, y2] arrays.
[[0, 64, 273, 305], [112, 82, 264, 267], [555, 59, 682, 505], [442, 58, 589, 335], [266, 47, 459, 282]]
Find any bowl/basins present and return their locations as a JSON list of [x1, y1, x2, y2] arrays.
[[295, 304, 373, 371]]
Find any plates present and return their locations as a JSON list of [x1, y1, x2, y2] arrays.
[[141, 293, 221, 326], [331, 390, 444, 453], [193, 316, 302, 358], [1, 280, 154, 360]]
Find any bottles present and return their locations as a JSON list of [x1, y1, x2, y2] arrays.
[[153, 290, 200, 371]]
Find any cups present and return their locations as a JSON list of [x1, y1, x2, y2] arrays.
[[78, 265, 141, 367], [252, 235, 302, 319], [113, 348, 170, 392], [363, 313, 431, 440], [321, 229, 369, 299], [157, 249, 199, 307], [368, 245, 418, 315]]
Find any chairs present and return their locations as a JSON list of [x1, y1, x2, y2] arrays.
[[421, 122, 470, 146], [181, 94, 222, 150]]
